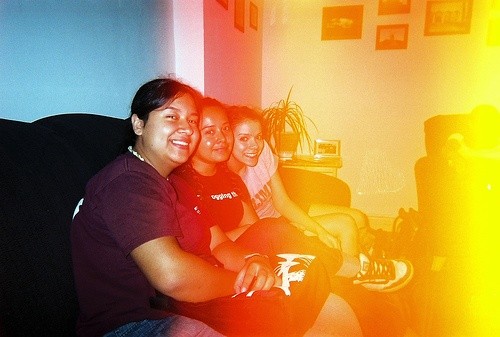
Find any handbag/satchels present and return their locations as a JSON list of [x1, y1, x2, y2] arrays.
[[182, 217, 331, 337]]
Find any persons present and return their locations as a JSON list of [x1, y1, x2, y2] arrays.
[[228, 105, 369, 258], [71, 78, 283, 337], [167, 96, 414, 337]]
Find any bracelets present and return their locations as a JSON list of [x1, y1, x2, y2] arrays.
[[243, 252, 270, 261]]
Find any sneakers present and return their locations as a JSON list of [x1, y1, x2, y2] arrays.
[[351, 254, 414, 293]]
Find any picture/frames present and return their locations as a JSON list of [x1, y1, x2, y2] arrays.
[[315, 140, 341, 157], [375, 25, 408, 49], [234, 0, 245, 33], [249, 2, 258, 30], [424, 0, 473, 35], [378, 0, 410, 15], [321, 6, 363, 40], [216, 0, 228, 10]]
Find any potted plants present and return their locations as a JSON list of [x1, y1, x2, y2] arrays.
[[262, 84, 319, 159]]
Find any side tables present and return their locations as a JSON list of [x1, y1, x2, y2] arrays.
[[279, 156, 343, 177]]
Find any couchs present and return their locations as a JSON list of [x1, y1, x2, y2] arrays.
[[0, 114, 350, 337]]
